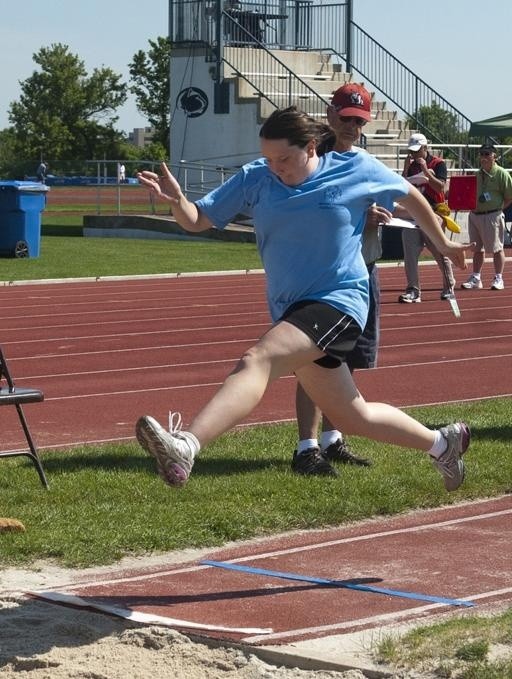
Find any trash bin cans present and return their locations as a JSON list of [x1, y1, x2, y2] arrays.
[[0, 180, 51, 260]]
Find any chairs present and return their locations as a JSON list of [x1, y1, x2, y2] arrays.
[[0, 350, 53, 493]]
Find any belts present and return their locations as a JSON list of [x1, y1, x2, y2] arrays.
[[471, 208, 503, 214]]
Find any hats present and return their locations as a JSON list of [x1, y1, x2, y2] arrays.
[[479, 144, 498, 153], [407, 133, 427, 152], [332, 83, 372, 122]]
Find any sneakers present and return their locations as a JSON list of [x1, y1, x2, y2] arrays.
[[135, 415, 194, 488], [399, 290, 422, 303], [462, 275, 484, 289], [441, 291, 456, 300], [430, 422, 470, 491], [491, 276, 504, 290], [291, 447, 341, 479], [319, 439, 372, 466]]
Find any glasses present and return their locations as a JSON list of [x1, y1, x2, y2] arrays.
[[480, 154, 491, 156], [336, 116, 366, 125]]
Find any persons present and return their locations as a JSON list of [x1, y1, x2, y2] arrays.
[[120, 163, 126, 184], [460, 142, 512, 291], [289, 82, 386, 480], [131, 104, 479, 488], [397, 133, 457, 305], [35, 162, 49, 184]]
